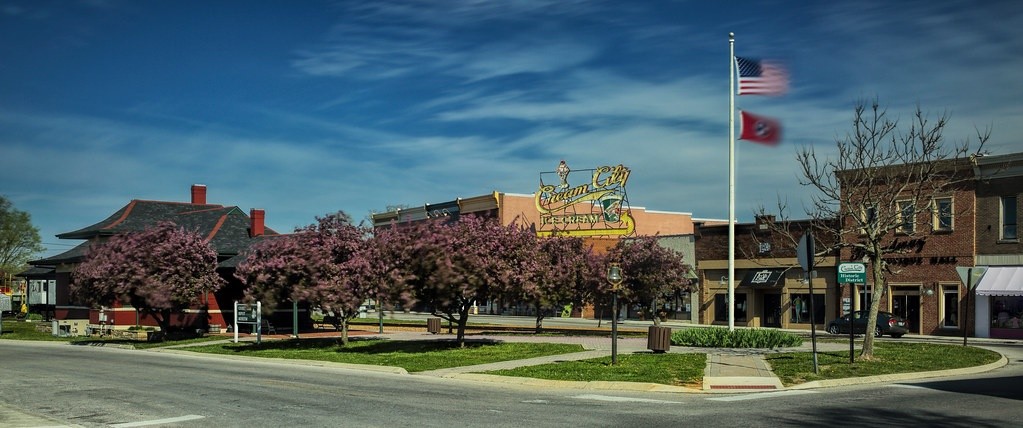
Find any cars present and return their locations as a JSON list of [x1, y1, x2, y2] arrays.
[[827, 310, 909, 337]]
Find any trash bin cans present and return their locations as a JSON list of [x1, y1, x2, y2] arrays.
[[647, 326, 671, 354], [427, 318, 441, 335]]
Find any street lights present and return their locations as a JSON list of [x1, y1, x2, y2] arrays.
[[606, 266, 622, 365]]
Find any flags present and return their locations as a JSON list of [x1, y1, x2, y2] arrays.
[[734, 56, 786, 95], [738, 108, 781, 146]]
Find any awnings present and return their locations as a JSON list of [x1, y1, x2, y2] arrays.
[[736, 270, 785, 288], [975, 266, 1023, 296]]
